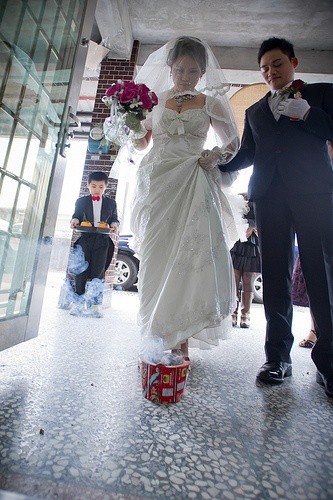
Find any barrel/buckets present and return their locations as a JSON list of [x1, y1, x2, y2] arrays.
[[140, 352, 190, 404]]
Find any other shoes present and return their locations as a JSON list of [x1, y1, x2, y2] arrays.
[[69, 303, 83, 315], [90, 304, 103, 318]]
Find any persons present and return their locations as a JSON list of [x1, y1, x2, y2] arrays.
[[103, 35, 241, 372], [70, 172, 120, 318], [230, 192, 261, 328], [291, 255, 317, 348], [200, 37, 333, 397]]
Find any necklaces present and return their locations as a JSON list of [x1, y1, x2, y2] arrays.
[[172, 94, 192, 113]]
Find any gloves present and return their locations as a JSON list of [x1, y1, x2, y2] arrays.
[[276, 96, 311, 120]]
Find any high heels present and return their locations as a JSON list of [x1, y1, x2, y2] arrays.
[[240, 308, 251, 327], [299, 329, 317, 348], [231, 308, 237, 327]]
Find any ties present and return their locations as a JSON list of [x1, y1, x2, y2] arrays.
[[271, 89, 286, 122]]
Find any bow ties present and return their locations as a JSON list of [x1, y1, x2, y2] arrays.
[[92, 196, 100, 201]]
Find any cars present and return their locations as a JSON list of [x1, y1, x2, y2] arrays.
[[112, 234, 263, 303]]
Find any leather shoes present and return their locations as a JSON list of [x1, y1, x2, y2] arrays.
[[256, 360, 292, 384], [316, 370, 333, 397]]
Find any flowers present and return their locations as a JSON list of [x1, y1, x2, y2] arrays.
[[278, 79, 308, 121], [101, 80, 158, 145]]
